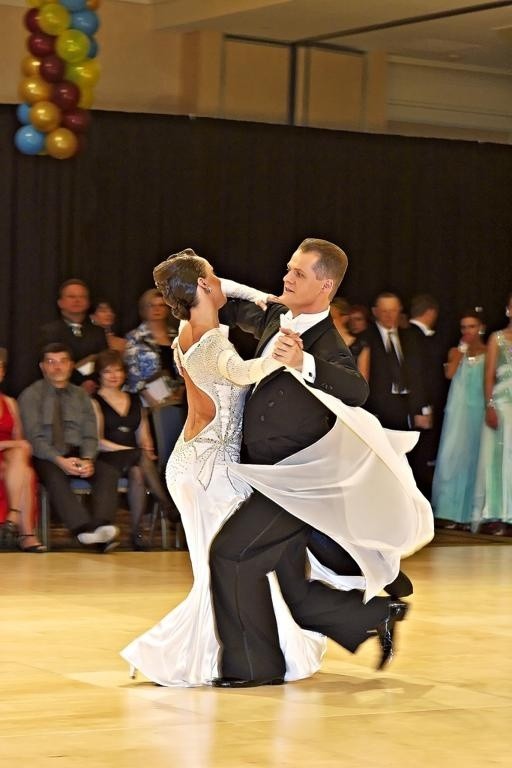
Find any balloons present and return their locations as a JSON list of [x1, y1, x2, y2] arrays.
[[12, 1, 101, 159]]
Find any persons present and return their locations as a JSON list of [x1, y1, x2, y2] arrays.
[[117, 249, 306, 686], [0, 279, 182, 553], [211, 238, 414, 687], [330, 290, 512, 537]]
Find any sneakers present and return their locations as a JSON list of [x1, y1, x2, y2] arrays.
[[77, 523, 121, 552]]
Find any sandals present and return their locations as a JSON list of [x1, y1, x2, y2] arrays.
[[0, 509, 46, 552]]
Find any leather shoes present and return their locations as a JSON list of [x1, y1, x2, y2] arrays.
[[377, 597, 406, 668], [212, 675, 284, 687]]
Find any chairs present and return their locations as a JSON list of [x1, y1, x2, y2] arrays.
[[39, 404, 186, 551]]
